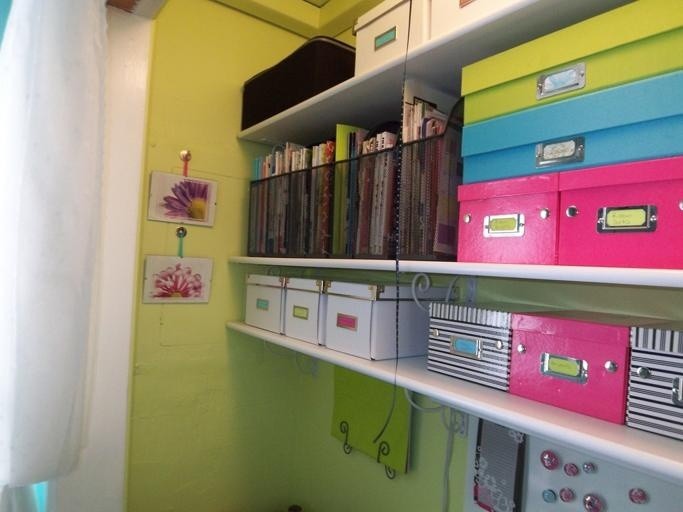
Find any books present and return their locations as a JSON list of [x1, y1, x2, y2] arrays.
[[251, 78, 463, 261]]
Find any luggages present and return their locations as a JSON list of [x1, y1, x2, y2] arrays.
[[242, 36, 355, 130]]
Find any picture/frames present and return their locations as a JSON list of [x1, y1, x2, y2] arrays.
[[149, 171, 219, 227], [142, 255, 213, 305]]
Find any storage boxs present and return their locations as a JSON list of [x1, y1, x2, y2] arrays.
[[428, 0, 516, 39], [511, 308, 673, 424], [428, 299, 573, 391], [626, 320, 683, 440], [352, 1, 430, 77], [461, 0, 683, 124], [282, 277, 325, 344], [326, 281, 460, 363], [245, 272, 284, 332], [558, 155, 683, 267], [457, 173, 557, 265], [462, 69, 683, 183]]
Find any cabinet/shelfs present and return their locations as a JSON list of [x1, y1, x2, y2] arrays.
[[229, 1, 683, 486]]
